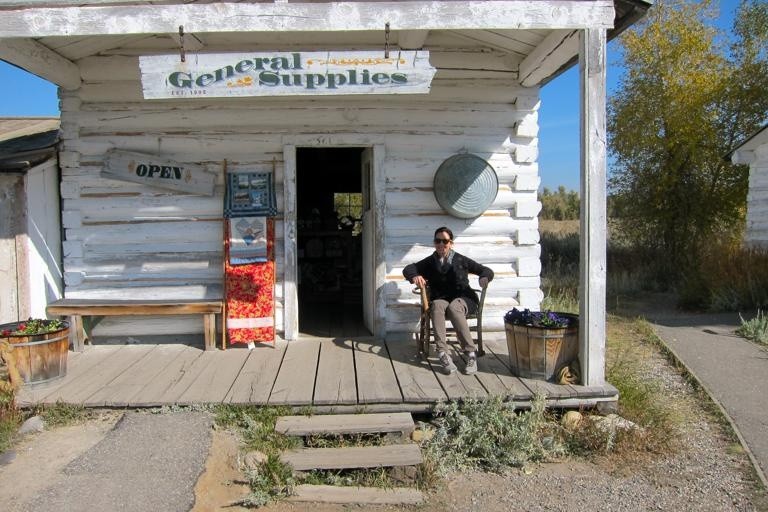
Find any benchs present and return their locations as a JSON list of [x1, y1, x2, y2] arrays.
[[48, 300, 223, 354]]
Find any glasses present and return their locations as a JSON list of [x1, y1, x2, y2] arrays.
[[434, 238, 452, 244]]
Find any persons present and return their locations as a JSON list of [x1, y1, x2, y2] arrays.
[[402, 226, 494, 375]]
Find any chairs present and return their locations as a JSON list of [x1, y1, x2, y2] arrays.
[[413, 280, 487, 358]]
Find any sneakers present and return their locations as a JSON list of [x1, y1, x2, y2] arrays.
[[464, 356, 477, 374], [440, 354, 457, 374]]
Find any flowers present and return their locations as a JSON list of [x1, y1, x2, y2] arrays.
[[2, 317, 63, 337], [505, 308, 573, 328]]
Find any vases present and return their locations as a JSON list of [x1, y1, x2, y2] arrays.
[[0, 320, 71, 387], [505, 321, 578, 380]]
